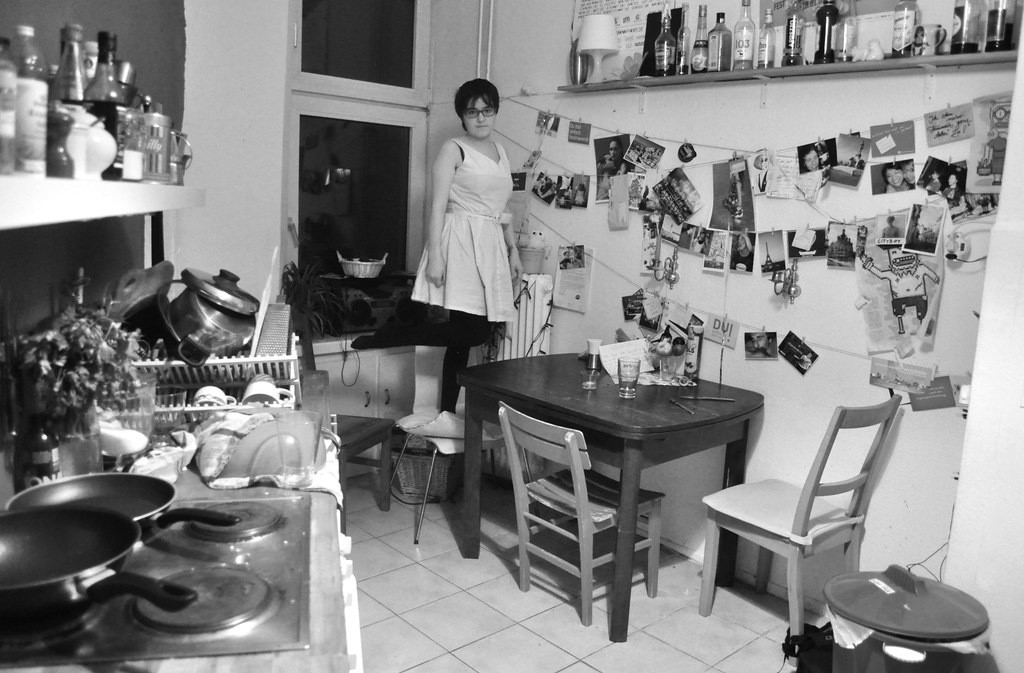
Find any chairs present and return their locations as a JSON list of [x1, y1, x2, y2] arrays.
[[332, 415, 394, 536], [497, 400, 667, 626], [390, 408, 504, 544], [701, 394, 902, 668]]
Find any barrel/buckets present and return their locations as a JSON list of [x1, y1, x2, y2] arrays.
[[519, 247, 546, 274]]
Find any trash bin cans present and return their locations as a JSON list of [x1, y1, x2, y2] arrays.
[[822, 564, 991, 672]]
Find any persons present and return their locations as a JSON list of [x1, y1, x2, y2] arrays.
[[730, 234, 754, 273], [411, 79, 523, 418], [560, 248, 584, 270], [745, 332, 777, 359], [798, 144, 820, 173], [882, 161, 916, 192], [926, 167, 963, 200], [595, 137, 631, 200], [881, 216, 899, 239]]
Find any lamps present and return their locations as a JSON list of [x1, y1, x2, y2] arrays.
[[577, 14, 619, 83]]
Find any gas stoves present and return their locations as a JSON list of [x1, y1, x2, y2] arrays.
[[1, 493, 364, 673]]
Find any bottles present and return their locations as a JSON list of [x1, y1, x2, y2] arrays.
[[0, 21, 194, 184], [58, 398, 105, 478], [655, 1, 1016, 78]]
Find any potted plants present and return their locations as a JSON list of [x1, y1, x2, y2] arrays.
[[280, 255, 352, 340]]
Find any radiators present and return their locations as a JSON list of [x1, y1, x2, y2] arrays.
[[487, 273, 553, 477]]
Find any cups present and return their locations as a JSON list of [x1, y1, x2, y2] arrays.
[[586, 338, 603, 370], [659, 358, 677, 382], [580, 370, 600, 390], [276, 409, 323, 488], [618, 357, 641, 399], [192, 373, 296, 423], [146, 385, 187, 436]]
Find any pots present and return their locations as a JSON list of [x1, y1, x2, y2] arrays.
[[4, 472, 242, 534], [167, 287, 257, 368], [0, 504, 198, 625]]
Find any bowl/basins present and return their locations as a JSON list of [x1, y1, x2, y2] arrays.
[[90, 373, 156, 459]]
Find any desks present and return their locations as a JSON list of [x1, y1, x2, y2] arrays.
[[457, 353, 764, 642]]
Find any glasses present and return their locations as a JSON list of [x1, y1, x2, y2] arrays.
[[464, 106, 495, 118]]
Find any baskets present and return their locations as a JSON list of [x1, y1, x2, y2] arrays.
[[333, 249, 388, 278], [391, 448, 487, 504]]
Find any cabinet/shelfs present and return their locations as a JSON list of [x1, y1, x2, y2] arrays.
[[0, 174, 364, 673], [295, 340, 416, 478]]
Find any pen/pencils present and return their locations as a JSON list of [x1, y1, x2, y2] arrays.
[[669, 397, 695, 415], [680, 395, 736, 401]]
